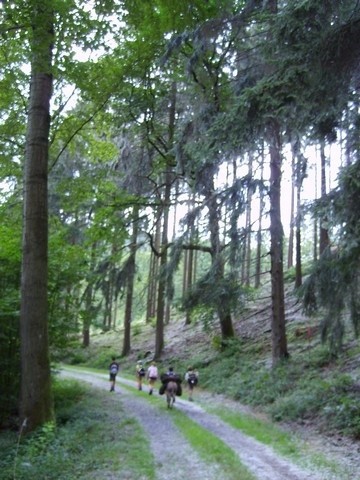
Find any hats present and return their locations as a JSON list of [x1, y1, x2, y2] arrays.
[[187, 365, 193, 371]]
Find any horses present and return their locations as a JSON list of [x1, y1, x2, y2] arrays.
[[164, 375, 178, 411]]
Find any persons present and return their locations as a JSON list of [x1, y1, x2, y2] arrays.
[[135, 360, 143, 390], [185, 366, 194, 402], [147, 362, 158, 395], [109, 356, 119, 392], [167, 367, 175, 376]]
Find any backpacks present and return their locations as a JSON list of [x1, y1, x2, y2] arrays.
[[111, 364, 117, 376], [149, 368, 157, 379], [188, 374, 197, 386], [139, 369, 144, 375]]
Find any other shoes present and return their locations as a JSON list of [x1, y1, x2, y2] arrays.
[[110, 389, 113, 392], [149, 389, 153, 395]]
